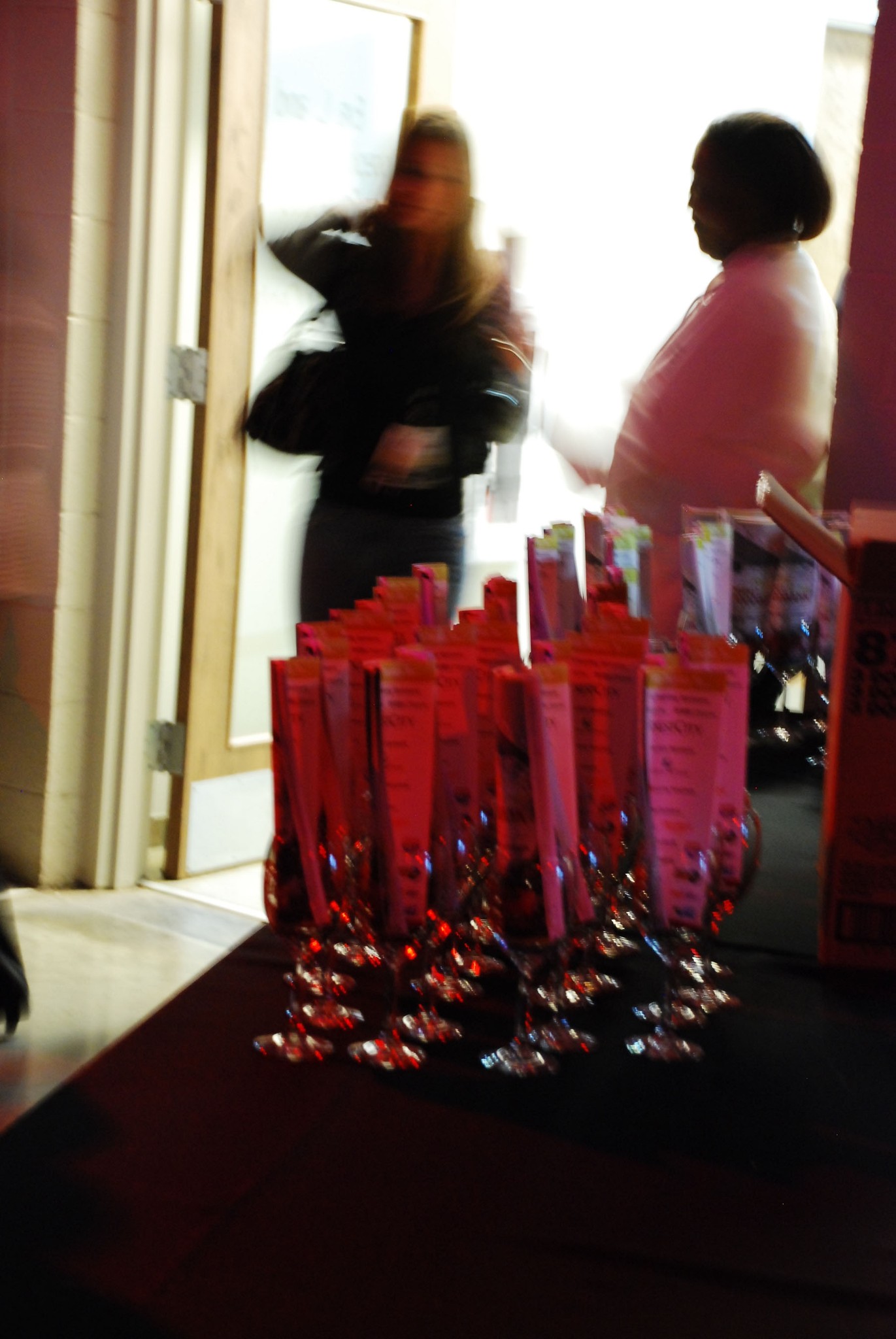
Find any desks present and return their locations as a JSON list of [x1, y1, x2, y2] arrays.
[[0, 784, 896, 1339]]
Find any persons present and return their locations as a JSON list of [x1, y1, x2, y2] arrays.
[[603, 118, 840, 590], [268, 110, 532, 624]]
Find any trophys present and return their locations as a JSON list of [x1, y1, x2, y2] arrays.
[[269, 511, 758, 1087]]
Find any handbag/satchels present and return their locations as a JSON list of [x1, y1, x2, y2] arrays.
[[243, 341, 344, 456]]
